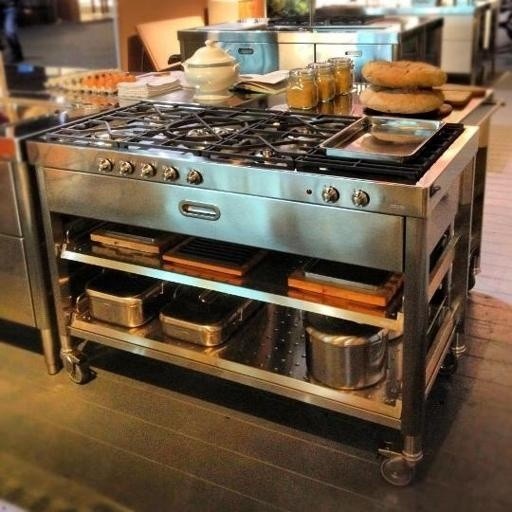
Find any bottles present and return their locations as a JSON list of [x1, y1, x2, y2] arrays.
[[286, 57, 354, 110]]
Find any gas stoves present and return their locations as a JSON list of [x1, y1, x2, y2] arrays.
[[28, 101, 480, 218], [267, 13, 383, 30]]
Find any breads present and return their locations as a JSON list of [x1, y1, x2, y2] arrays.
[[369, 132, 426, 144], [361, 59, 448, 90], [359, 85, 446, 114]]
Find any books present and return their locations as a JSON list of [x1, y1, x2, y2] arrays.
[[227, 70, 292, 95]]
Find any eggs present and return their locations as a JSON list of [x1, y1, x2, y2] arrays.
[[81, 73, 137, 90]]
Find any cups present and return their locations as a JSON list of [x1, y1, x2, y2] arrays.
[[182, 39, 240, 101]]
